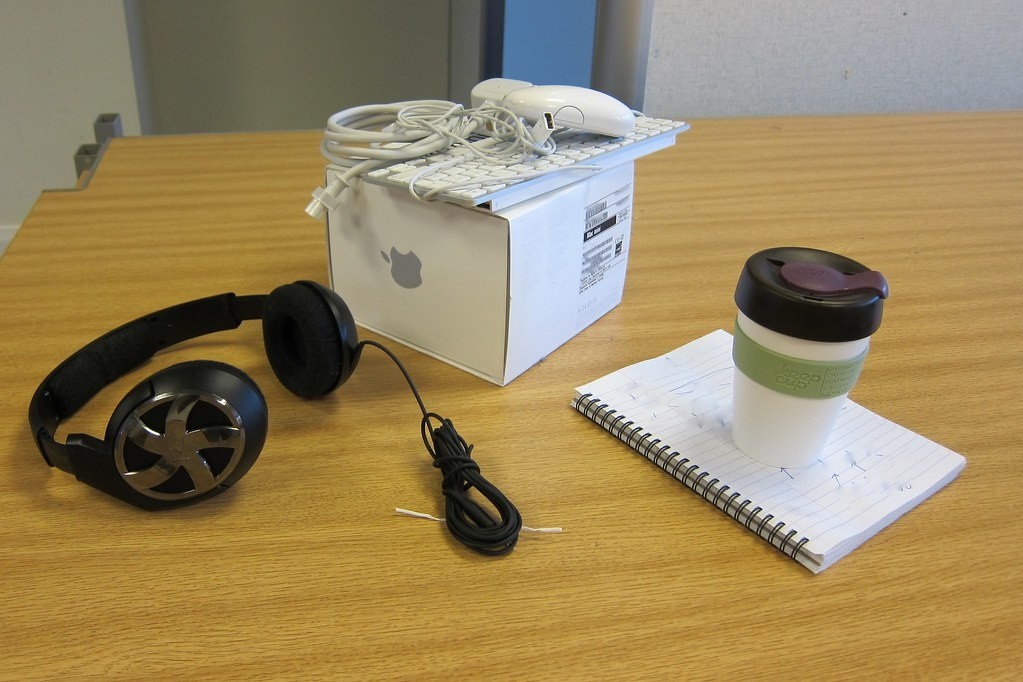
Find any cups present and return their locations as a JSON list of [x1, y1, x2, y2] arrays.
[[730, 246, 889, 466]]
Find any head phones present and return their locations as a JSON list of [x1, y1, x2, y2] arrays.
[[29, 280, 360, 514]]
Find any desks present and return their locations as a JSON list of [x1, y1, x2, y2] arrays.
[[0, 110, 1023, 682]]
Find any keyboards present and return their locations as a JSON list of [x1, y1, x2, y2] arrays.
[[359, 116, 692, 207]]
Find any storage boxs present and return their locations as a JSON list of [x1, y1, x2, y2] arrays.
[[323, 142, 633, 388]]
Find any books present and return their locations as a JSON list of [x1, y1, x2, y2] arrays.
[[569, 328, 967, 577]]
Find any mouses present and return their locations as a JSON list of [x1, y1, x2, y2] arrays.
[[502, 86, 637, 136]]
